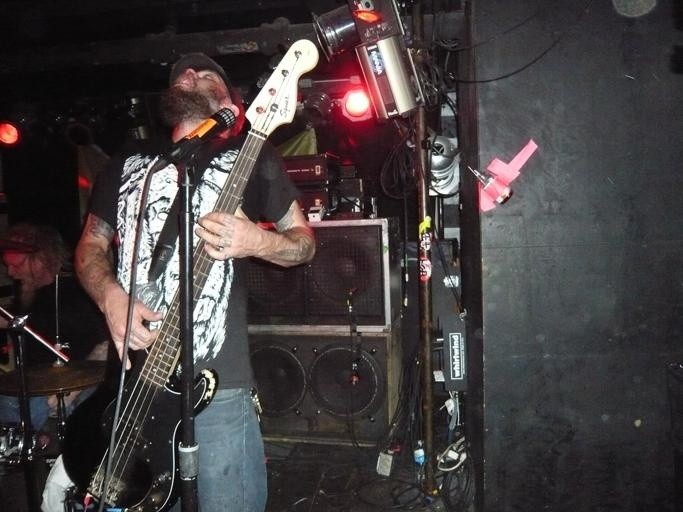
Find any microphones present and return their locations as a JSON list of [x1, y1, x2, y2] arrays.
[[153, 107, 236, 175]]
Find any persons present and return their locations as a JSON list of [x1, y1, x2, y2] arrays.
[[0, 217, 110, 435]]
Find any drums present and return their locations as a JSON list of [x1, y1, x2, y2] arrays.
[[0, 423, 38, 465]]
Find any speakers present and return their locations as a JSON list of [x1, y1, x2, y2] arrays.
[[246, 217, 403, 334], [247, 319, 404, 448]]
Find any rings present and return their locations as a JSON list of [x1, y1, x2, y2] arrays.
[[215, 237, 222, 247]]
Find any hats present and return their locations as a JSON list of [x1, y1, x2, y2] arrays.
[[169, 52, 246, 138]]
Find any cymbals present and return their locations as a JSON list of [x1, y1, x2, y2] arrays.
[[0, 360, 114, 397]]
